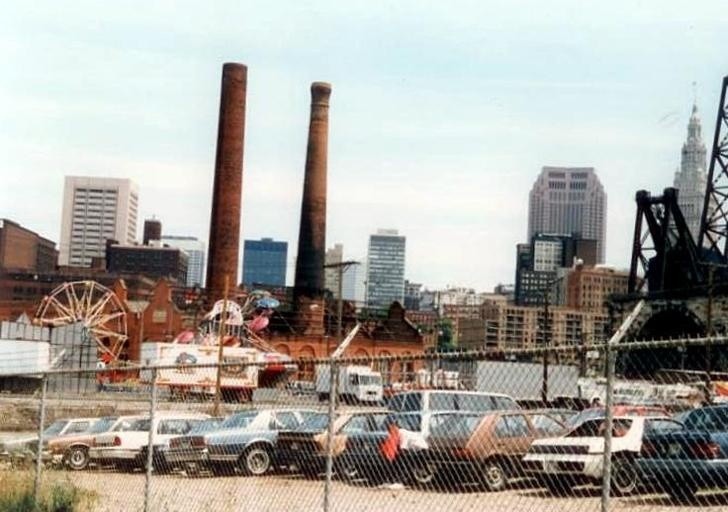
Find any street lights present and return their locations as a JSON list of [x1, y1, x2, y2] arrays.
[[308, 303, 335, 356], [190, 279, 207, 334], [511, 230, 596, 407]]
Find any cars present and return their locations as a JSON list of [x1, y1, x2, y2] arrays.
[[1, 416, 103, 470], [23, 416, 121, 472], [340, 410, 460, 486], [274, 410, 397, 480], [159, 415, 233, 477], [88, 416, 207, 475], [411, 409, 572, 492], [635, 404, 728, 500], [162, 408, 321, 477], [521, 416, 685, 497]]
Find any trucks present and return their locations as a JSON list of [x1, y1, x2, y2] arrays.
[[382, 361, 728, 412], [314, 363, 383, 407]]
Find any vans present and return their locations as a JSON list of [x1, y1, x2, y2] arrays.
[[381, 392, 520, 450]]
